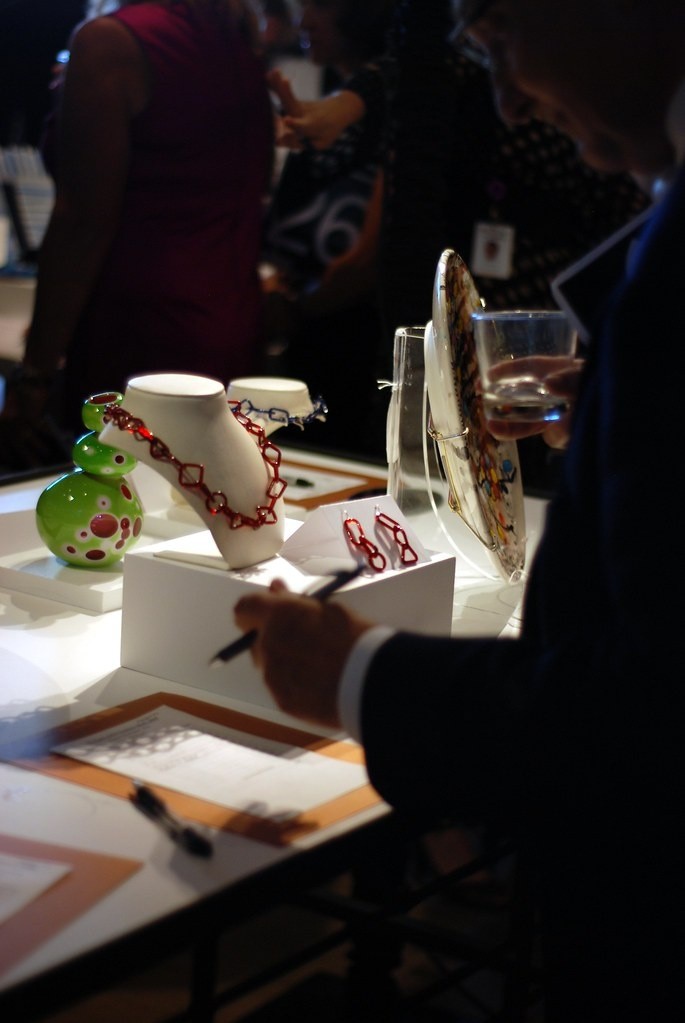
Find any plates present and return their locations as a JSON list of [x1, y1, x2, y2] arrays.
[[424, 248, 528, 587]]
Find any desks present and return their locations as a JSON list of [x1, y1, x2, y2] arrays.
[[0, 441, 551, 1023]]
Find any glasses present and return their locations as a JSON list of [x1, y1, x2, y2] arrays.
[[445, 0, 502, 73]]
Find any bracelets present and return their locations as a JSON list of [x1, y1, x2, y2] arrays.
[[11, 361, 50, 390]]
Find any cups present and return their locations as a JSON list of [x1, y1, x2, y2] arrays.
[[474, 311, 577, 423], [386, 325, 469, 579]]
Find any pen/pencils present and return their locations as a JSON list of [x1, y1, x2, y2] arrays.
[[205, 560, 367, 673], [130, 776, 214, 861]]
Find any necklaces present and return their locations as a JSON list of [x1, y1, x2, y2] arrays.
[[103, 400, 288, 529]]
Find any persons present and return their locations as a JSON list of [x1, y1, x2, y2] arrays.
[[234, 0, 685, 1023], [0, 0, 279, 474]]
